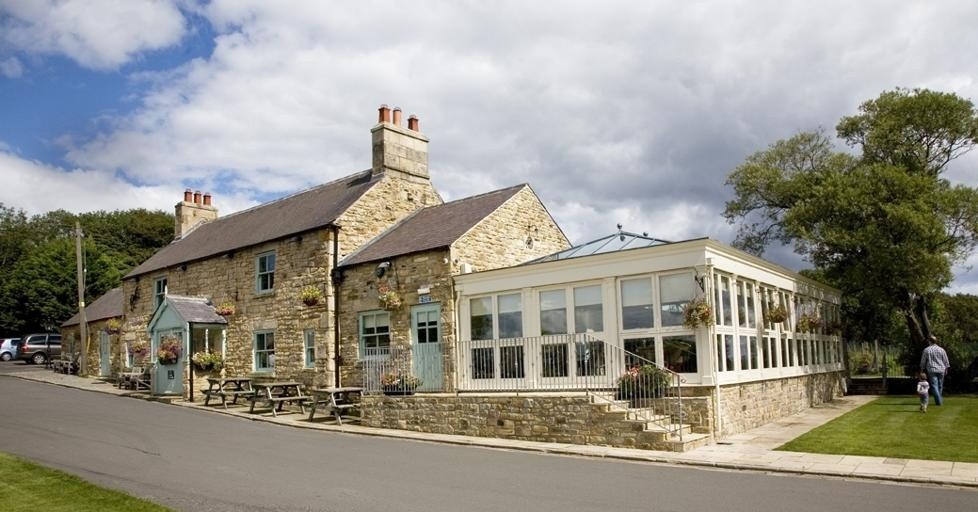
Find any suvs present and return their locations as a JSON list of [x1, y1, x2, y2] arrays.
[[15, 333, 62, 365]]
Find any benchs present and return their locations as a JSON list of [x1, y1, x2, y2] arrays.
[[301, 397, 344, 421], [243, 392, 309, 418], [199, 387, 255, 408], [331, 403, 362, 426]]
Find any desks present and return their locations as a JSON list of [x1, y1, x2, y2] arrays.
[[250, 381, 304, 417], [307, 387, 366, 424], [205, 377, 253, 407]]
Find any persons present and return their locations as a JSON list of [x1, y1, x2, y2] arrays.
[[920, 334, 950, 406], [916, 373, 930, 413]]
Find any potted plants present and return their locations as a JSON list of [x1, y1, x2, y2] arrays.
[[214, 301, 236, 316], [104, 318, 224, 372], [375, 285, 402, 311], [617, 364, 673, 398], [683, 295, 715, 330], [764, 303, 787, 323], [299, 285, 322, 307]]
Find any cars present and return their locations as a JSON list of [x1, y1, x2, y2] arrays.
[[0, 338, 22, 361]]
